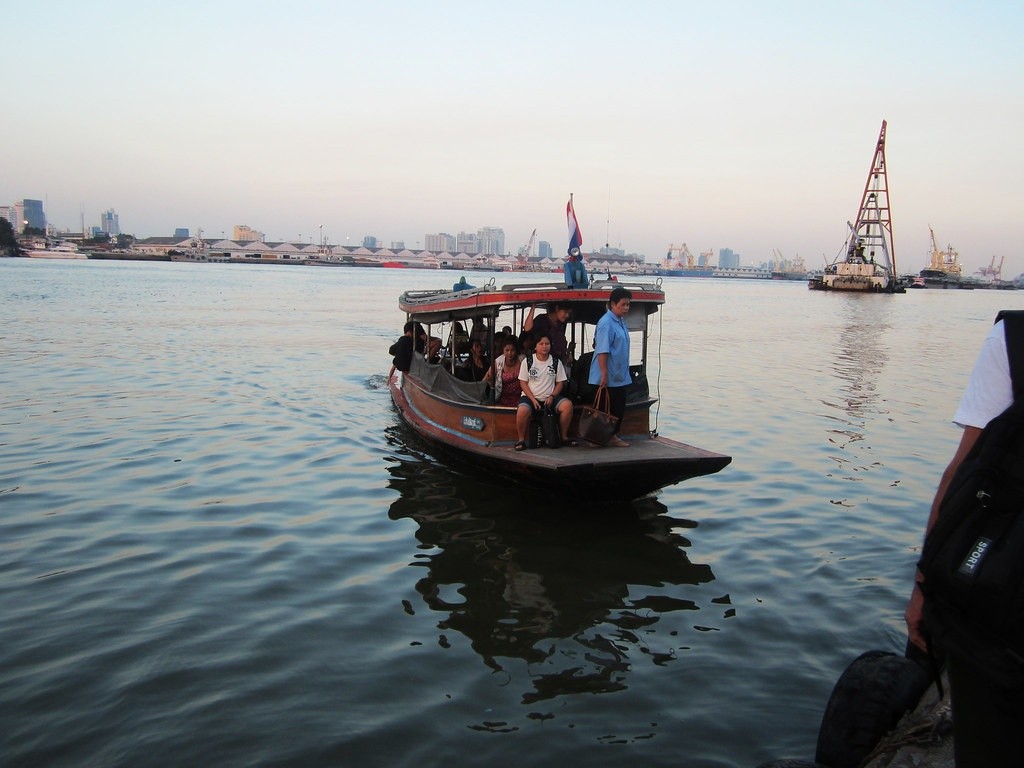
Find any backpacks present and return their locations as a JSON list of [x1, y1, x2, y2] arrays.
[[919, 307, 1024, 689]]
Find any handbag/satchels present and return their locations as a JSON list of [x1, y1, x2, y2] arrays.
[[528, 403, 562, 449], [576, 387, 619, 447]]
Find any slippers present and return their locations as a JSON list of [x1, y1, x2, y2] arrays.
[[563, 437, 578, 447], [515, 441, 526, 450]]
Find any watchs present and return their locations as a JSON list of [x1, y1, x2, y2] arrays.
[[549, 394, 556, 400]]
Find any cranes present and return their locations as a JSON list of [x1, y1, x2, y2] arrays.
[[981, 255, 1006, 283], [663, 240, 717, 270], [771, 247, 807, 273]]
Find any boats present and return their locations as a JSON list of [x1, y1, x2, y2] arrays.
[[386, 189, 735, 507], [918, 224, 975, 289], [805, 115, 909, 293], [910, 276, 928, 289]]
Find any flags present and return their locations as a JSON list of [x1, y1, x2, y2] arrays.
[[567, 193, 583, 262]]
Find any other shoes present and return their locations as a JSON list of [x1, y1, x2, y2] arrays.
[[611, 439, 630, 447]]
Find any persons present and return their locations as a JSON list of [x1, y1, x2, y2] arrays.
[[386, 302, 594, 407], [514, 332, 579, 451], [907, 309, 1024, 768], [588, 287, 632, 446]]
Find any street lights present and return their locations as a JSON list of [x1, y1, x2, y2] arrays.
[[317, 224, 324, 249]]
